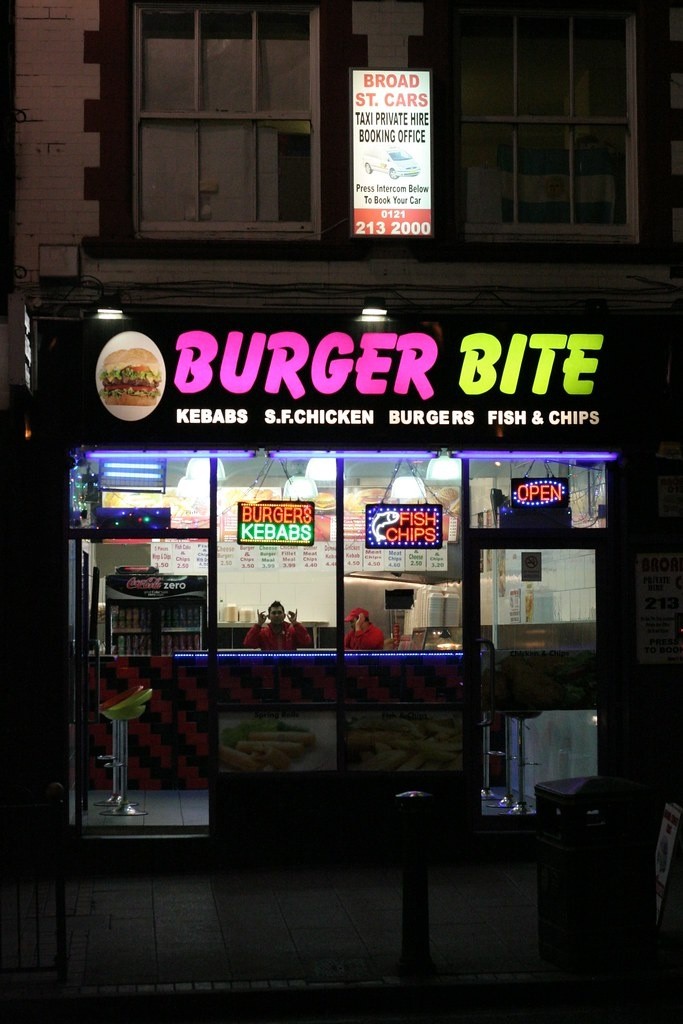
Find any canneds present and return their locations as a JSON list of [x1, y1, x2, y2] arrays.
[[110, 604, 200, 655]]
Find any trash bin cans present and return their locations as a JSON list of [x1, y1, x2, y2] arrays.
[[533, 774, 652, 967]]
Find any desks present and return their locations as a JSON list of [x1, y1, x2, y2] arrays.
[[217, 620, 330, 650]]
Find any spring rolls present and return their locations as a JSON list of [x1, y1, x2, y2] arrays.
[[218, 729, 317, 773]]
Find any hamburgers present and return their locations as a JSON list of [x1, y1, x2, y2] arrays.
[[98, 348, 160, 406]]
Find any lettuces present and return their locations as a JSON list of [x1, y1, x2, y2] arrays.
[[219, 717, 283, 750]]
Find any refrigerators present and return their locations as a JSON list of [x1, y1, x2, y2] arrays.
[[104, 565, 207, 655]]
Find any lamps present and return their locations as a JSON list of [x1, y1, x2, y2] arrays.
[[176, 477, 211, 498], [186, 457, 226, 481], [390, 476, 426, 499], [361, 296, 388, 316], [101, 462, 163, 470], [96, 295, 124, 314], [262, 445, 442, 459], [80, 444, 260, 458], [305, 457, 346, 482], [101, 472, 164, 479], [284, 460, 318, 499], [425, 448, 461, 480], [445, 448, 619, 460]]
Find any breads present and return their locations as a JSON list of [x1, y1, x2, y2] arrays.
[[344, 717, 463, 771]]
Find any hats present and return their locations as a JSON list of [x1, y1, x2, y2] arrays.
[[344, 608, 369, 622]]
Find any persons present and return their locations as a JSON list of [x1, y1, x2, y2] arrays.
[[243, 601, 311, 650], [344, 607, 384, 650]]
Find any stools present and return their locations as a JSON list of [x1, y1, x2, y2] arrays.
[[479, 709, 509, 801], [99, 688, 152, 815], [495, 710, 541, 814], [94, 686, 144, 806]]
